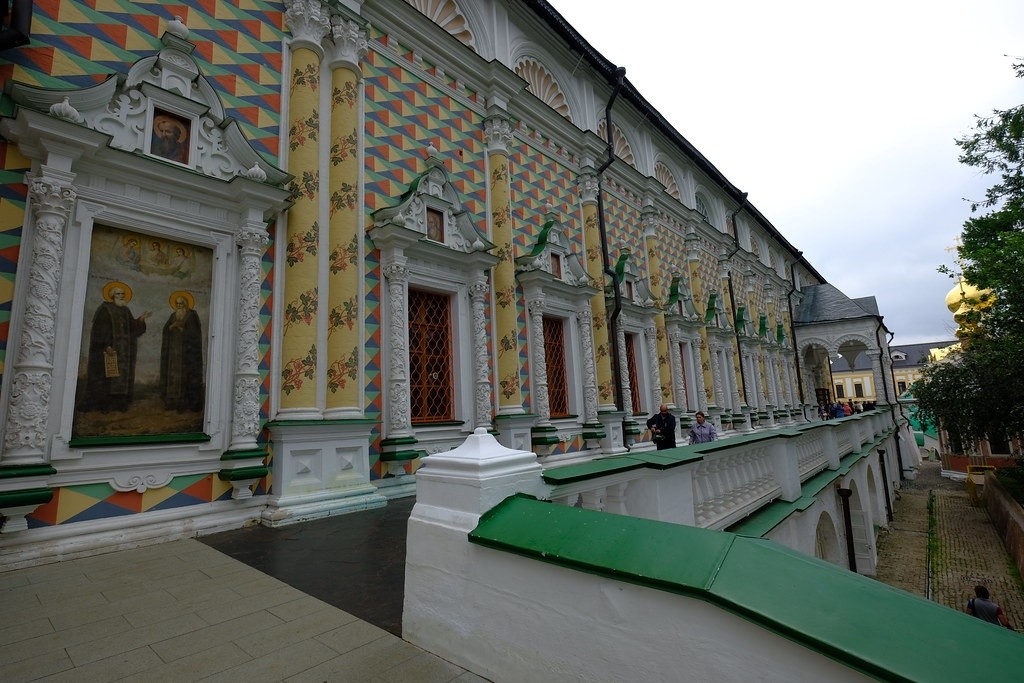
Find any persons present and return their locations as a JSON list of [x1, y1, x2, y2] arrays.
[[818, 399, 876, 421], [966, 585, 1015, 631], [646, 404, 676, 451], [152, 114, 189, 166], [689, 411, 718, 444], [79, 238, 204, 413]]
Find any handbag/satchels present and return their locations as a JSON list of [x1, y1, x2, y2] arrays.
[[650, 432, 665, 445], [823, 413, 827, 416], [818, 412, 821, 417]]
